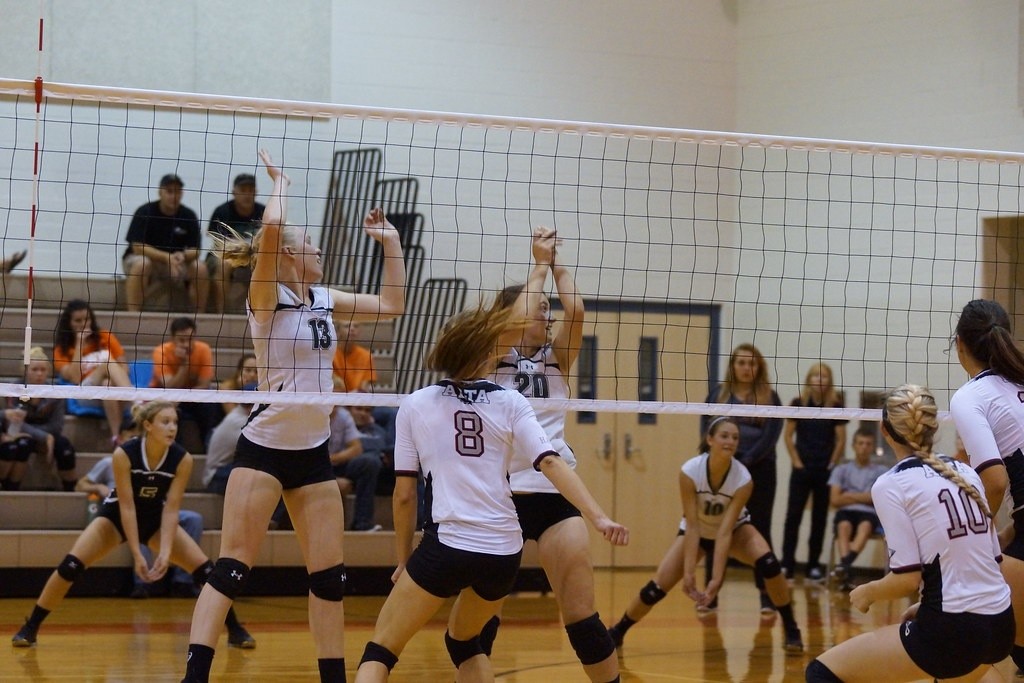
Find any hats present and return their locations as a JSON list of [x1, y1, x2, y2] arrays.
[[233, 173, 256, 188], [160, 173, 186, 187]]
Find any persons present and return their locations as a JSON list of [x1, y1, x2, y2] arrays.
[[11, 399, 256, 650], [0, 173, 399, 599], [804, 384, 1015, 683], [780, 363, 850, 585], [178, 147, 406, 683], [480, 224, 624, 683], [826, 427, 892, 580], [694, 344, 783, 620], [942, 298, 1024, 678], [351, 303, 629, 683], [609, 414, 804, 654]]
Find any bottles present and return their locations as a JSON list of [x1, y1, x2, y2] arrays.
[[8, 402, 28, 437], [86, 493, 100, 523]]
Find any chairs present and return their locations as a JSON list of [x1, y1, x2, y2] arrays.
[[829, 454, 890, 584]]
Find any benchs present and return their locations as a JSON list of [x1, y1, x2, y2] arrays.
[[0, 269, 542, 565]]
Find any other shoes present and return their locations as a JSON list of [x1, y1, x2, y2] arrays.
[[760, 597, 776, 615], [779, 565, 798, 581], [607, 626, 624, 649], [228, 625, 257, 649], [695, 594, 718, 611], [782, 628, 805, 657], [802, 566, 827, 583], [832, 562, 854, 596], [11, 623, 38, 647]]
